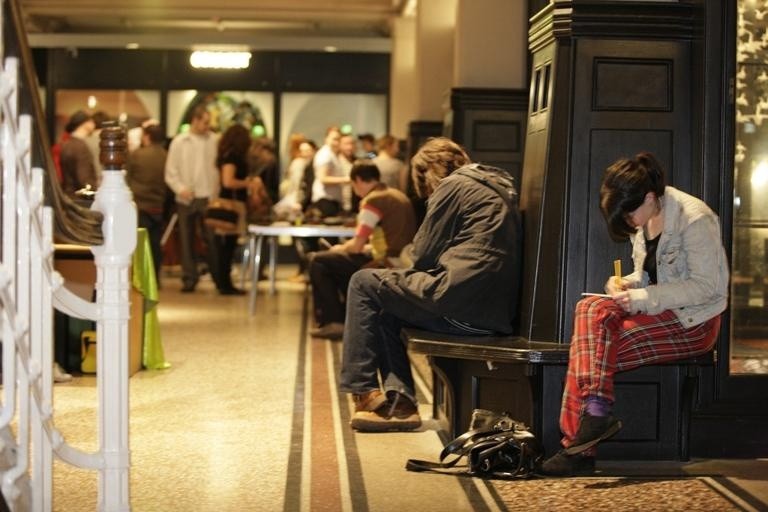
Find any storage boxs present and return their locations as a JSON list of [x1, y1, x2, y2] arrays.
[[81, 328, 97, 374]]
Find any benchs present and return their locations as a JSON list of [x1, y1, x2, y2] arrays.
[[396, 325, 726, 474]]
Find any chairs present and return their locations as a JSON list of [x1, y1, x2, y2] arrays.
[[159, 199, 247, 249]]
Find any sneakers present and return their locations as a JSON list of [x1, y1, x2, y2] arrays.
[[349, 389, 389, 426], [352, 391, 422, 430], [566, 409, 622, 456], [534, 449, 595, 476], [309, 322, 344, 340]]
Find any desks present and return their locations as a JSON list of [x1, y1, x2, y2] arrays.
[[245, 225, 358, 319], [54, 228, 147, 378]]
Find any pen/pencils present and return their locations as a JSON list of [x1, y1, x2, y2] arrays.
[[614, 260, 621, 287]]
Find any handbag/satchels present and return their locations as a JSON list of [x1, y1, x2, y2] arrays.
[[405, 409, 536, 481]]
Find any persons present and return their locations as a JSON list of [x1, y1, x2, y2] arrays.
[[306, 159, 415, 341], [122, 102, 279, 296], [536, 150, 731, 478], [287, 125, 408, 275], [339, 138, 523, 431], [59, 111, 105, 197]]
